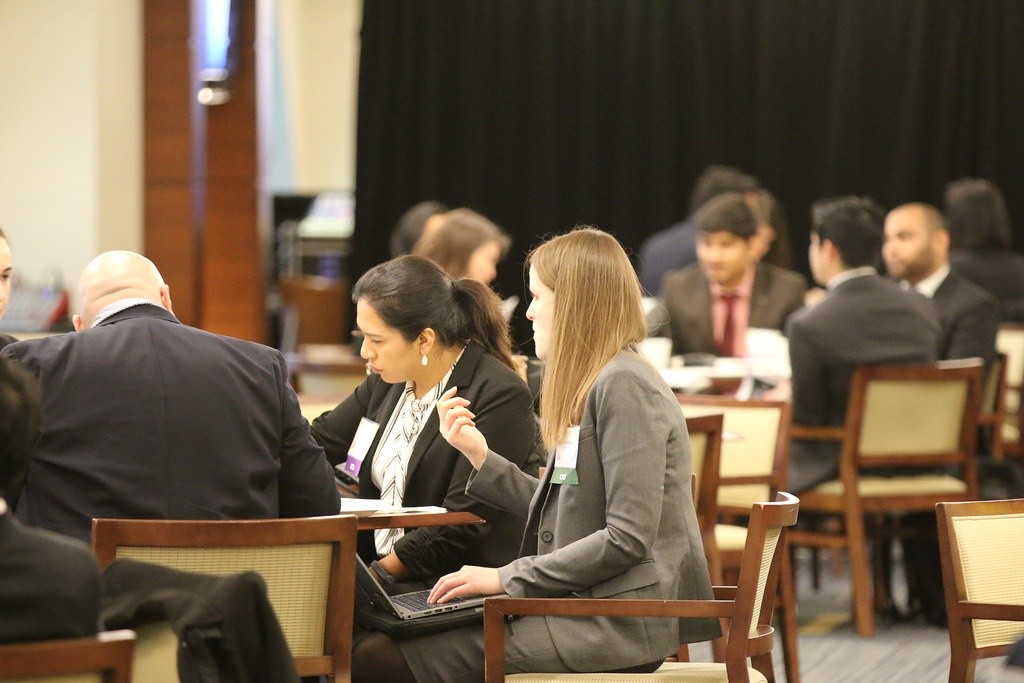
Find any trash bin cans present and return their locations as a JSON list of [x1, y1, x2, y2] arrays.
[[277, 238, 353, 344]]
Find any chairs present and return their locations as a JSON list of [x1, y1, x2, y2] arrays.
[[0, 323, 1024, 683]]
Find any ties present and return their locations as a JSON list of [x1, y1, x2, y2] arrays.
[[721, 294, 738, 357]]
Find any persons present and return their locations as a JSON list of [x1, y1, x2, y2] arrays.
[[0, 227, 20, 356], [785, 176, 1024, 631], [351, 229, 723, 683], [0, 353, 106, 646], [388, 200, 520, 323], [311, 255, 536, 584], [0, 250, 341, 547], [638, 165, 805, 366]]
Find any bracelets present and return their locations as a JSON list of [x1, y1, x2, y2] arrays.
[[370, 560, 397, 584]]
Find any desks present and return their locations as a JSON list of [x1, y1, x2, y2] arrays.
[[338, 510, 488, 530]]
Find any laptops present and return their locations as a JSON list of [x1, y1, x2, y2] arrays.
[[352, 552, 492, 621]]
